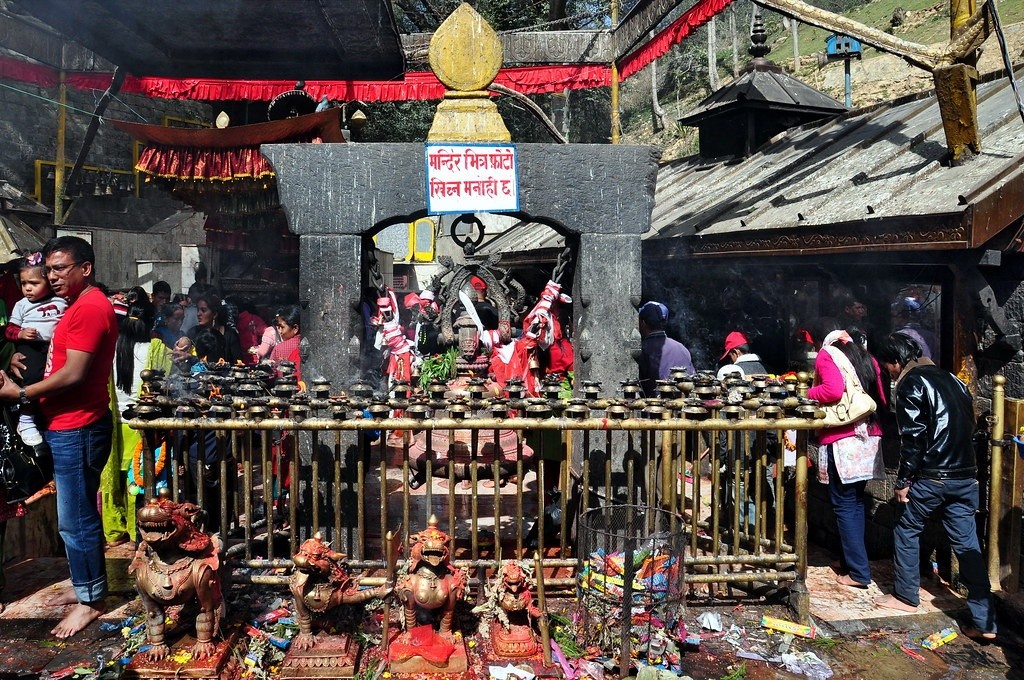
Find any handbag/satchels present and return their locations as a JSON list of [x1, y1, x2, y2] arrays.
[[812, 346, 877, 428]]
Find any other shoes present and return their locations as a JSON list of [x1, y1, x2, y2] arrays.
[[17, 422, 43, 446]]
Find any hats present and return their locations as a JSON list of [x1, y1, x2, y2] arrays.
[[639, 301, 669, 327], [891, 297, 926, 320], [720, 332, 748, 361]]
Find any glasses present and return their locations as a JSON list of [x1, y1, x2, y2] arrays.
[[790, 330, 814, 344], [41, 261, 87, 278]]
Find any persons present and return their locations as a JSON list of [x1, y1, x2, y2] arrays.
[[470, 276, 499, 331], [0, 235, 299, 639], [637, 296, 999, 641]]
